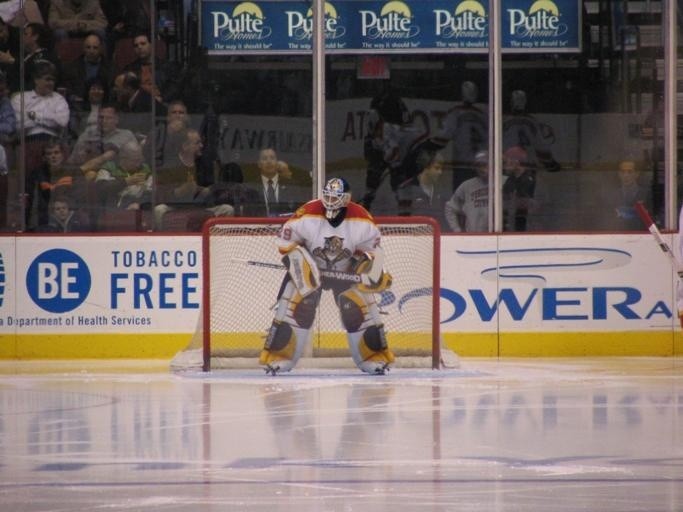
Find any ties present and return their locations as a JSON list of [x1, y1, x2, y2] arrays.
[[266, 178, 278, 207]]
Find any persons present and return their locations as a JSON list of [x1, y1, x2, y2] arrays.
[[243, 148, 300, 218], [600, 161, 654, 234], [260, 178, 393, 375], [0, 1, 236, 232], [360, 82, 561, 232]]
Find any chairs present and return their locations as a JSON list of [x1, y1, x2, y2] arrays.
[[84, 202, 212, 235]]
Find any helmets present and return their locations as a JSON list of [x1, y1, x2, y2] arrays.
[[320, 176, 351, 211], [460, 81, 479, 103], [510, 91, 527, 111]]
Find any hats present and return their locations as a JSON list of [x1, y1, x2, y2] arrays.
[[472, 151, 488, 166], [504, 146, 526, 161]]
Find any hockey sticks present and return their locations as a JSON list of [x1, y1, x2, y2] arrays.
[[230, 245, 385, 286]]
